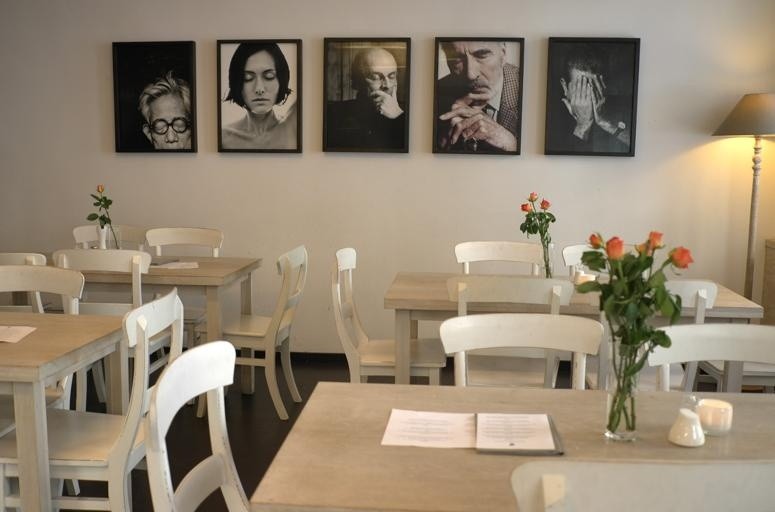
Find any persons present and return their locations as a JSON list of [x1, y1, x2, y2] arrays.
[[221, 42, 298, 151], [550, 45, 632, 154], [138, 71, 192, 151], [327, 46, 406, 149]]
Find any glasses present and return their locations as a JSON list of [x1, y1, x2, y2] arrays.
[[145, 116, 194, 135]]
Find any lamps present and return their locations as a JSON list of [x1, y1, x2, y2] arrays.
[[712, 92, 775, 299]]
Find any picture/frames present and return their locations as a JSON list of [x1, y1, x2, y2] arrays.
[[111, 40, 199, 154], [216, 38, 303, 153], [543, 36, 642, 158], [323, 36, 409, 154]]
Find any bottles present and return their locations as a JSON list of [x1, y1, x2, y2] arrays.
[[668, 406, 706, 447], [574, 270, 584, 286]]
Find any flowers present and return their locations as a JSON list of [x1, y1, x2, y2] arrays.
[[520, 193, 554, 278], [86, 183, 120, 248]]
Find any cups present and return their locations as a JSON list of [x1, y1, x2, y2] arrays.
[[697, 398, 734, 438], [581, 274, 595, 284]]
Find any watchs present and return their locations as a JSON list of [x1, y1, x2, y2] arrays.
[[612, 119, 629, 141]]
